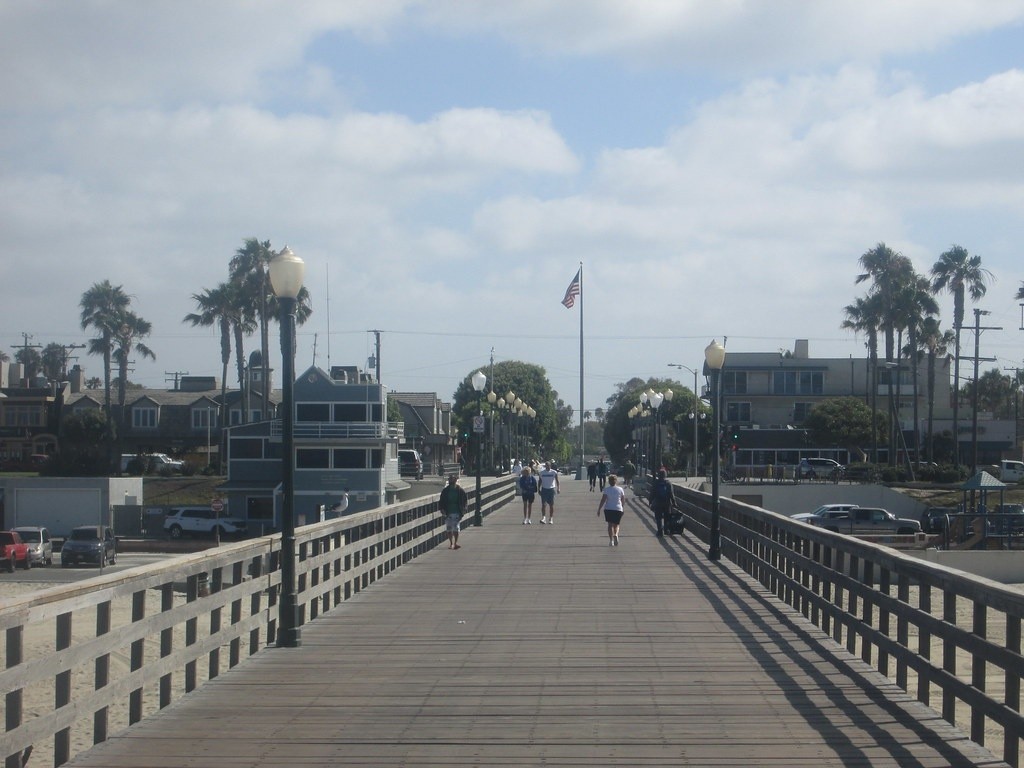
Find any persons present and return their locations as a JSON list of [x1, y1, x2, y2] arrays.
[[596, 458, 608, 492], [597, 474, 626, 546], [439, 473, 468, 550], [518, 466, 538, 525], [587, 459, 597, 492], [550, 459, 558, 471], [624, 459, 637, 489], [511, 461, 523, 477], [649, 469, 678, 538], [537, 462, 561, 525]]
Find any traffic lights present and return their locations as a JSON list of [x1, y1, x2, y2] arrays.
[[731, 445, 737, 451], [730, 426, 740, 444]]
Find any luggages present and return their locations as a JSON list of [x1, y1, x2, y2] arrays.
[[669, 506, 685, 536]]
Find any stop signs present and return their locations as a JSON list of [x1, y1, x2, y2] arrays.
[[211, 498, 223, 511]]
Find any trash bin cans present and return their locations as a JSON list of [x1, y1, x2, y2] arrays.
[[633, 477, 646, 497], [576, 467, 587, 480]]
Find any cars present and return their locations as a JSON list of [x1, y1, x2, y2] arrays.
[[163, 506, 247, 540], [798, 458, 845, 482]]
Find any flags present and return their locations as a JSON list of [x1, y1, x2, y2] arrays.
[[561, 268, 581, 311]]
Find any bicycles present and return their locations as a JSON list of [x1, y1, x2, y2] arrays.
[[720, 466, 746, 483]]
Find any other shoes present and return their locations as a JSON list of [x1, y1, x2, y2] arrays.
[[549, 517, 554, 524], [540, 518, 546, 524], [609, 541, 614, 546], [454, 544, 461, 549], [614, 535, 618, 545], [448, 545, 453, 549], [528, 519, 532, 524], [522, 519, 527, 524], [664, 529, 670, 535], [656, 530, 663, 537]]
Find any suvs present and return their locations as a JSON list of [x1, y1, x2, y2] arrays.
[[397, 448, 425, 482], [60, 522, 118, 567], [0, 526, 54, 573]]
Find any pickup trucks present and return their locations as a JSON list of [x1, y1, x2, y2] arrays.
[[976, 460, 1024, 483], [807, 507, 922, 536], [787, 504, 894, 524], [120, 453, 184, 478]]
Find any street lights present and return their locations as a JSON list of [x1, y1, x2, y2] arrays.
[[268, 246, 303, 649], [471, 370, 487, 527], [884, 359, 979, 522], [486, 388, 538, 476], [668, 363, 698, 477], [704, 339, 724, 560], [626, 386, 674, 484]]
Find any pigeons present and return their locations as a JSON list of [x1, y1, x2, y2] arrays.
[[322, 492, 349, 517]]
